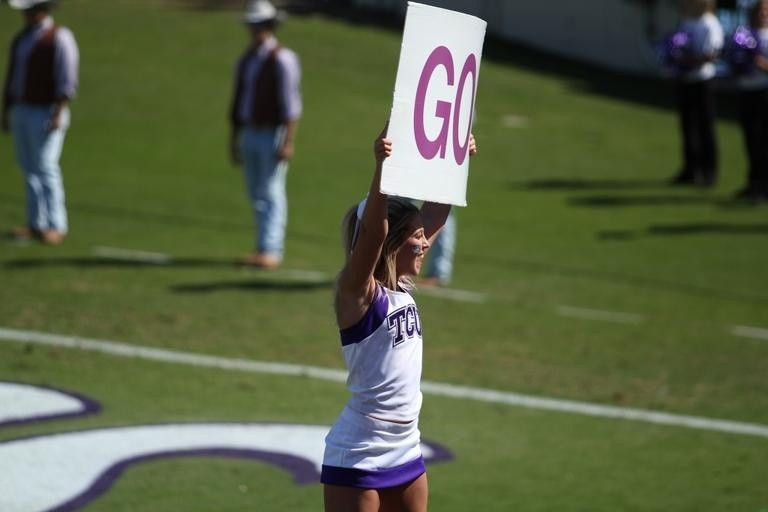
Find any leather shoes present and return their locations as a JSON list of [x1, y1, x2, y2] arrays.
[[9, 226, 39, 238], [42, 230, 63, 243], [236, 252, 278, 267]]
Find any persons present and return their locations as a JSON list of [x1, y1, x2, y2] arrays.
[[661, 2, 728, 190], [227, 0, 304, 271], [428, 201, 461, 288], [0, 0, 81, 247], [717, 0, 768, 199], [317, 112, 479, 510]]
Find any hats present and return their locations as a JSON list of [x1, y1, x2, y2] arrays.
[[8, 0, 48, 9], [241, 0, 287, 25]]
